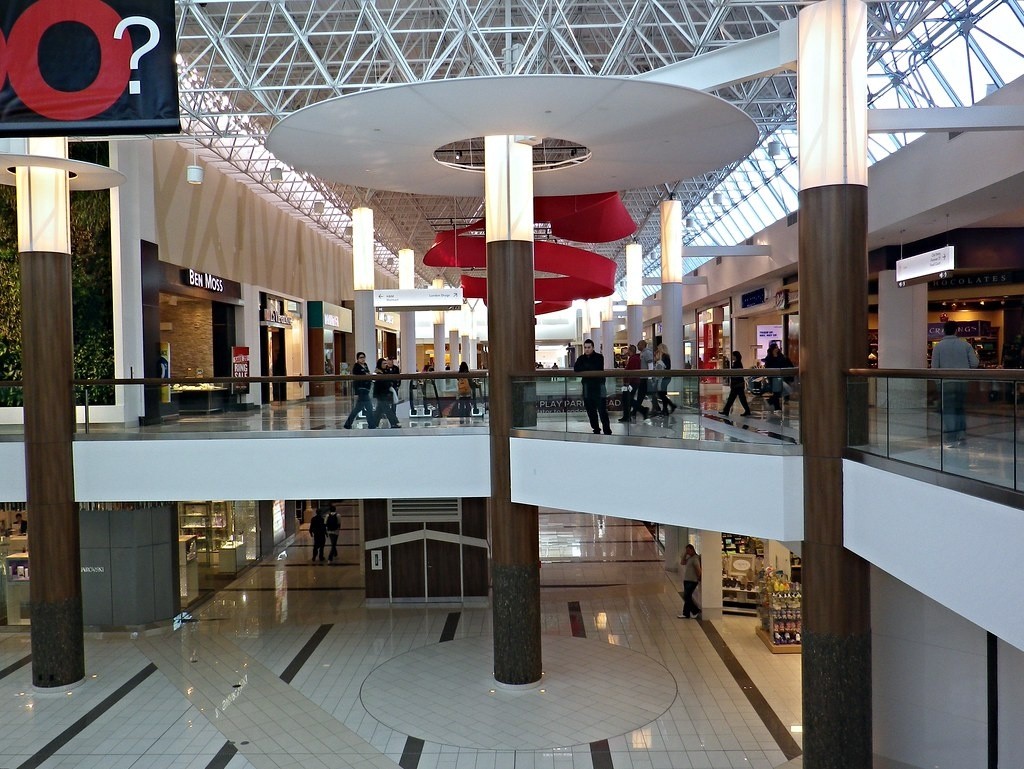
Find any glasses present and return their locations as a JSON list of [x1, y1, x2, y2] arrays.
[[360, 357, 365, 358]]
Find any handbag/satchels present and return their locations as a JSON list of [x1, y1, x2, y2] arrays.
[[391, 387, 399, 404], [965, 382, 982, 408], [648, 379, 661, 391]]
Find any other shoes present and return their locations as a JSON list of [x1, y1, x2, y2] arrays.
[[328, 560, 331, 564], [618, 416, 631, 422], [692, 613, 698, 618], [677, 615, 686, 617], [741, 411, 751, 416], [670, 404, 677, 413], [958, 440, 967, 447], [642, 407, 649, 419], [946, 441, 958, 448], [312, 558, 315, 561], [784, 400, 788, 404], [650, 408, 661, 417], [661, 409, 669, 416], [719, 411, 729, 416], [334, 554, 337, 557], [629, 411, 636, 417], [320, 557, 325, 561]]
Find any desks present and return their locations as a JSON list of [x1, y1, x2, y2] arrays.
[[171, 389, 228, 415]]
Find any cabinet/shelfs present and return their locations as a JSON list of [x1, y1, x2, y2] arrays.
[[0, 533, 30, 625], [180, 502, 229, 563], [721, 533, 801, 654]]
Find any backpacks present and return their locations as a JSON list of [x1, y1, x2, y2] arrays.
[[327, 513, 339, 531], [458, 378, 471, 394]]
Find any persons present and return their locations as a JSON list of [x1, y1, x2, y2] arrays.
[[535, 362, 543, 369], [574, 338, 612, 435], [718, 351, 750, 416], [676, 544, 701, 619], [372, 356, 401, 428], [343, 352, 377, 429], [12, 513, 27, 535], [324, 506, 341, 565], [931, 320, 981, 448], [456, 362, 481, 424], [445, 363, 450, 370], [422, 357, 434, 372], [617, 340, 677, 422], [698, 358, 703, 363], [552, 363, 559, 382], [764, 344, 795, 417], [308, 509, 326, 566]]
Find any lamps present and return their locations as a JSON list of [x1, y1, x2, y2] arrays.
[[270, 160, 393, 267], [651, 131, 778, 260], [186, 111, 204, 184]]
